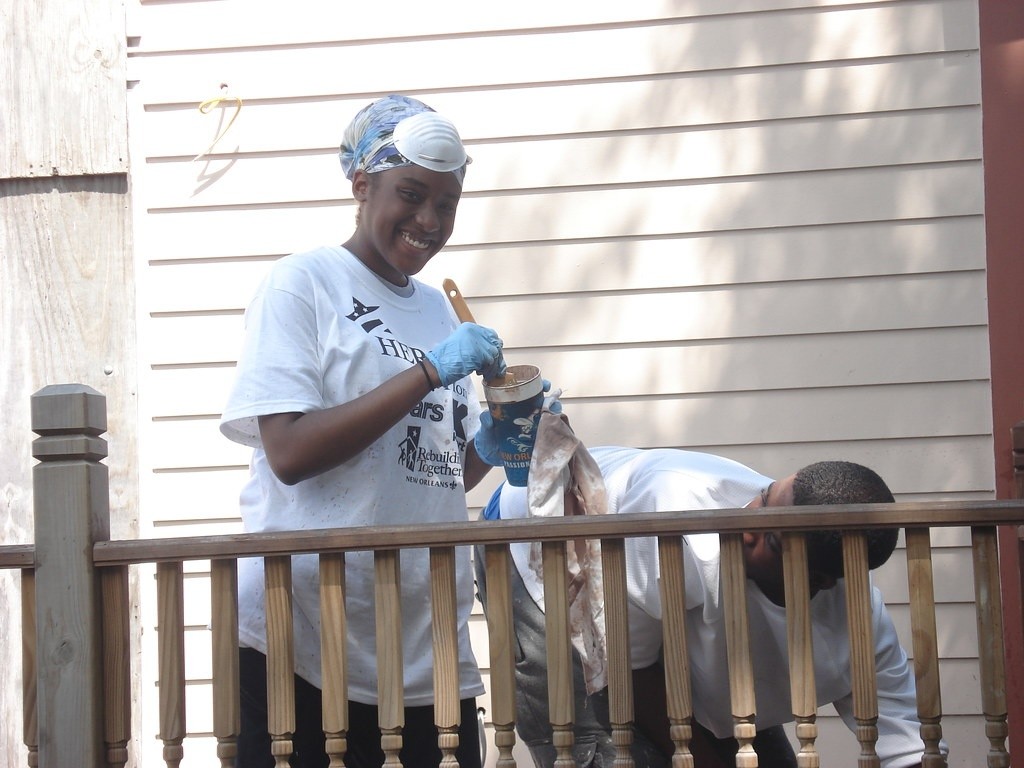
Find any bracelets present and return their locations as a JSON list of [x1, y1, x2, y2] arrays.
[[418, 360, 435, 391]]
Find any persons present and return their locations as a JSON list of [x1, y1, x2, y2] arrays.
[[219, 94, 562, 768], [474, 445, 949, 768]]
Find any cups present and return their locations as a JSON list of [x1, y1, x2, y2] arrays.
[[480, 365, 547, 487]]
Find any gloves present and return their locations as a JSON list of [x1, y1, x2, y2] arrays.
[[426, 322, 508, 383], [474, 378, 563, 467]]
